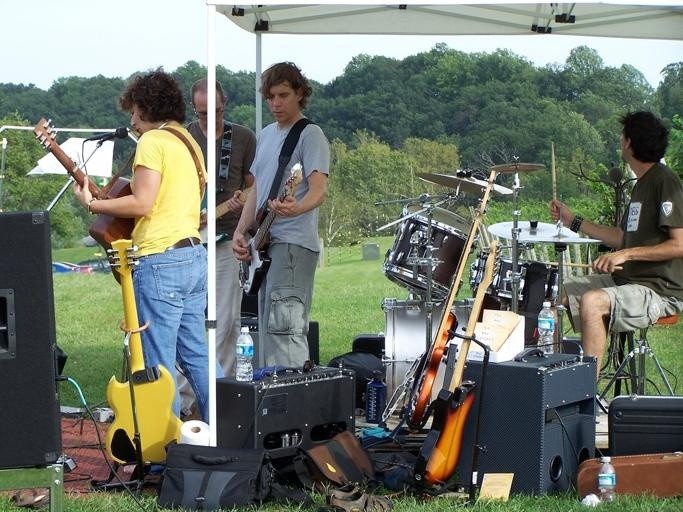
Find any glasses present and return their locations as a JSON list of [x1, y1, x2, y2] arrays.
[[191, 103, 224, 119]]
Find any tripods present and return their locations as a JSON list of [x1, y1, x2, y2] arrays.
[[93, 347, 163, 506], [601, 337, 674, 397]]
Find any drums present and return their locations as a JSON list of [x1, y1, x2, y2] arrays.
[[383, 300, 477, 430], [384, 204, 479, 298]]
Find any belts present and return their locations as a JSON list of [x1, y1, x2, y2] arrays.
[[165, 237, 200, 250]]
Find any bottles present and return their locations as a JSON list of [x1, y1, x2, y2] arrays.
[[537, 300, 555, 358], [596, 456, 616, 505], [365, 369, 389, 423], [235, 325, 255, 382]]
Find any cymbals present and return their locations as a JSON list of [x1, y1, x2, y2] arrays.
[[491, 165, 545, 173], [519, 236, 602, 245], [487, 221, 578, 241], [415, 172, 513, 197], [375, 193, 449, 205]]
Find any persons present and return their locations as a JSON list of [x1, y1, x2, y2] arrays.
[[176, 78, 257, 424], [231, 61, 331, 376], [551, 111, 683, 378], [70, 71, 225, 479]]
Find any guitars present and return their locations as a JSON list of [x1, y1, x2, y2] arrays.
[[107, 240, 185, 465], [414, 241, 501, 486], [199, 186, 251, 245], [403, 169, 495, 432], [32, 118, 135, 285], [239, 163, 302, 295]]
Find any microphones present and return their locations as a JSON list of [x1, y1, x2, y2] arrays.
[[87, 127, 129, 141]]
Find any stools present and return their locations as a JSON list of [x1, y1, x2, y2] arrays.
[[596, 315, 678, 415]]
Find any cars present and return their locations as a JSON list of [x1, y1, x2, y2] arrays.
[[52, 257, 111, 273]]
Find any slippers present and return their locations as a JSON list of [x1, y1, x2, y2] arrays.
[[9, 488, 50, 510]]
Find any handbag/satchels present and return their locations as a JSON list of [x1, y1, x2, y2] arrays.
[[369, 451, 423, 492], [157, 439, 260, 510], [295, 431, 380, 501]]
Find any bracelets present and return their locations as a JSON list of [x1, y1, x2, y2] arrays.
[[570, 216, 583, 232], [87, 197, 96, 215]]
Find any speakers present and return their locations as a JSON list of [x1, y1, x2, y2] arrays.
[[216, 365, 357, 458], [240, 318, 319, 370], [458, 348, 598, 495], [607, 394, 683, 459], [0, 211, 69, 470]]
